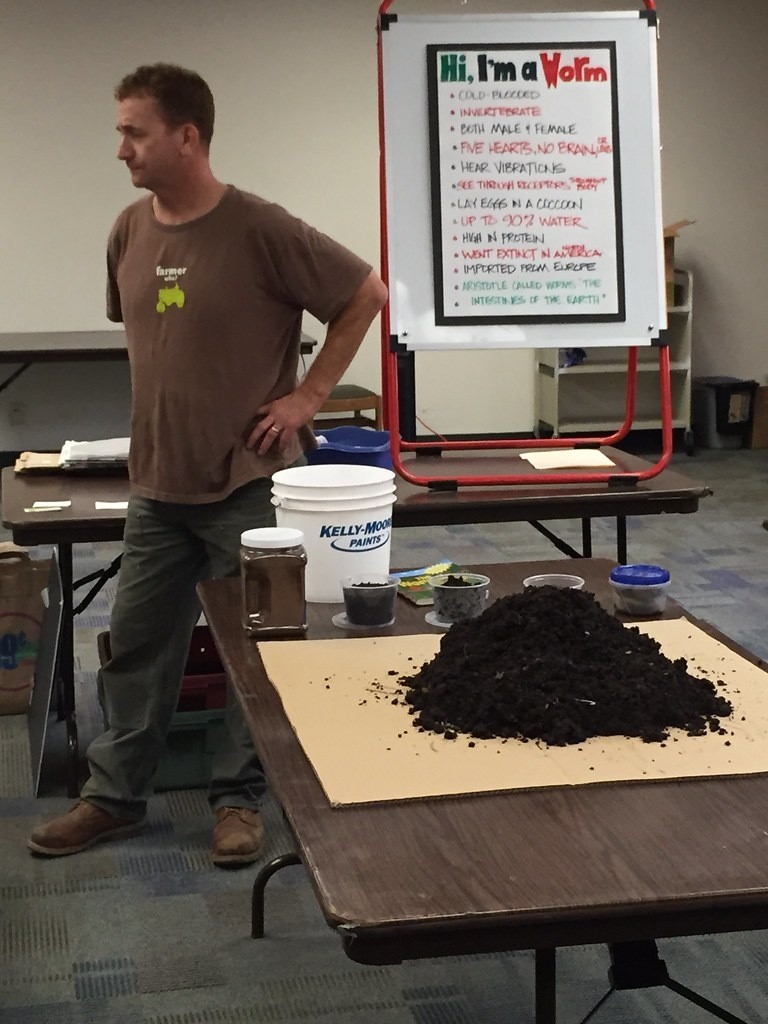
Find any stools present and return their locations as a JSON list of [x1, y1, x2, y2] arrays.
[[308, 383, 385, 431]]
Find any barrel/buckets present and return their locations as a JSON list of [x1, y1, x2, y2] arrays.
[[270, 464, 397, 604]]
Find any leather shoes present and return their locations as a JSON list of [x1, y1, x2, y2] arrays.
[[27, 797, 148, 856], [209, 805, 267, 866]]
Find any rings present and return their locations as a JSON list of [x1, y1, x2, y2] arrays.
[[271, 426, 280, 433]]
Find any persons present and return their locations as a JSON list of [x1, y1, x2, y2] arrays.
[[25, 61, 387, 863]]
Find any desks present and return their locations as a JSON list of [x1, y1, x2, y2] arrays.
[[196, 558, 768, 1024], [0, 330, 317, 393], [0, 445, 712, 801]]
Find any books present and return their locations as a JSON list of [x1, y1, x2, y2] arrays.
[[389, 557, 488, 605]]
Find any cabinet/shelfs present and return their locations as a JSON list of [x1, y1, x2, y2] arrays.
[[533, 267, 697, 460]]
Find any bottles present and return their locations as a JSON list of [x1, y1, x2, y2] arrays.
[[238, 527, 309, 639]]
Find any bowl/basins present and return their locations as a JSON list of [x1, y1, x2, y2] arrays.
[[427, 572, 490, 624], [304, 425, 402, 470], [339, 572, 401, 625], [522, 573, 586, 591], [608, 564, 672, 616]]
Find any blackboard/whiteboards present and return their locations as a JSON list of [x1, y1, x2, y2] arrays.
[[428, 40, 628, 327]]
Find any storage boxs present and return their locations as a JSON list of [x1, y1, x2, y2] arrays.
[[97, 625, 227, 792], [0, 541, 54, 716], [307, 426, 402, 470]]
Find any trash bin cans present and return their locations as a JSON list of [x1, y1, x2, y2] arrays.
[[691, 376, 760, 450]]
